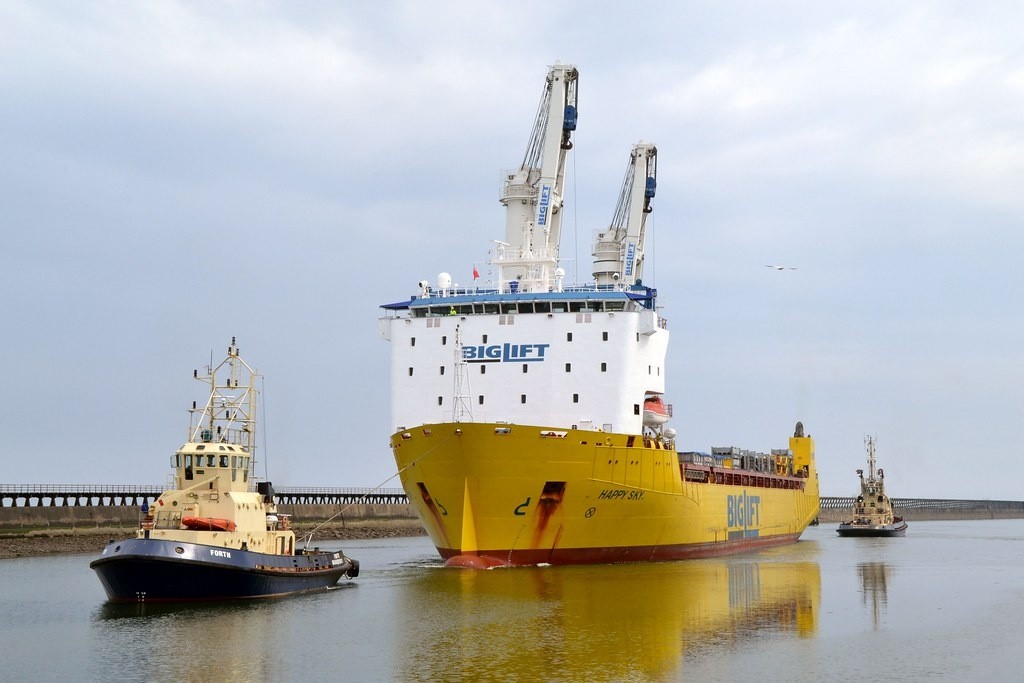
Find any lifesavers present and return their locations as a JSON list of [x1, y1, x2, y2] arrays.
[[282, 516, 288, 528], [200, 429, 213, 442], [347, 560, 359, 578], [857, 519, 861, 523]]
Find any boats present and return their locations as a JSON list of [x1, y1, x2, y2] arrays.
[[377, 53, 821, 568], [89, 334, 361, 618], [835, 434, 908, 538]]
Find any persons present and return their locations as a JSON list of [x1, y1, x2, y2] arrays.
[[645, 431, 662, 441], [450, 307, 456, 316]]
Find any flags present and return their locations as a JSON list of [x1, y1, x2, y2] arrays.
[[473, 269, 480, 280]]
